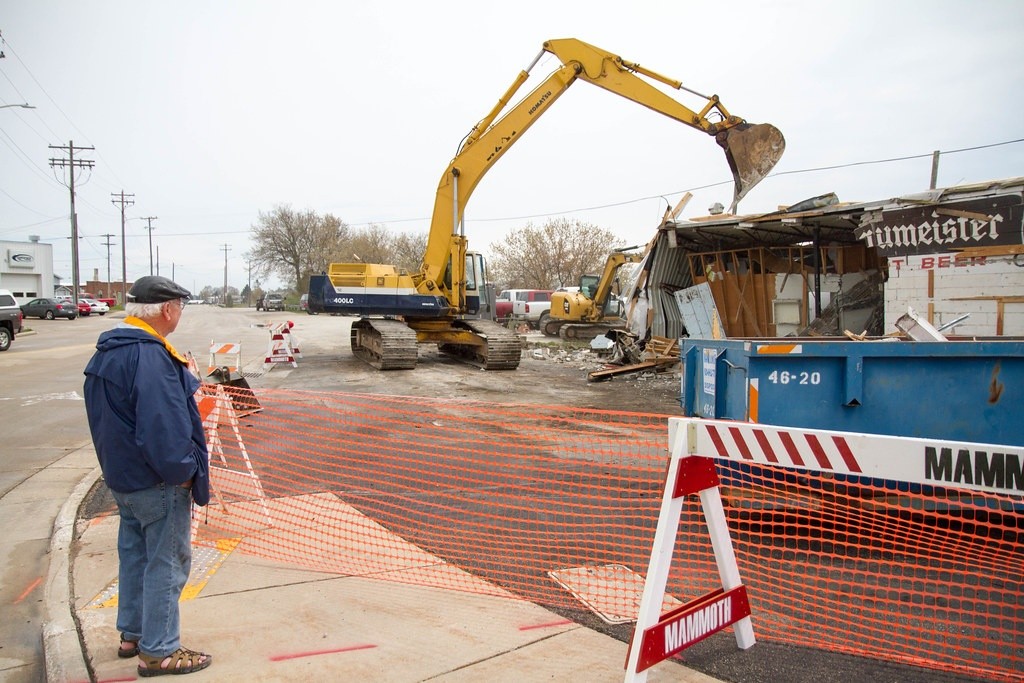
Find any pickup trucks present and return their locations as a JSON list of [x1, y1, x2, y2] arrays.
[[496, 290, 536, 302], [493, 290, 557, 323], [512, 286, 629, 329], [78, 293, 117, 308]]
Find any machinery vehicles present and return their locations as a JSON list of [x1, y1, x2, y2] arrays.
[[540, 248, 694, 342], [305, 38, 787, 371]]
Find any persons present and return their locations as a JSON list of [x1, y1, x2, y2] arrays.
[[83, 276, 213, 676]]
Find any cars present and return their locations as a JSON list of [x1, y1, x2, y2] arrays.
[[300, 293, 308, 311], [19, 298, 78, 321], [79, 299, 110, 316], [262, 293, 286, 312], [60, 299, 91, 317], [256, 294, 266, 311], [0, 289, 23, 352]]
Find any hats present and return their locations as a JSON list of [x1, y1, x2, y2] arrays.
[[126, 276, 191, 304]]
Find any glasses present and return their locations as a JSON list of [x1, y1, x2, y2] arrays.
[[168, 301, 186, 310]]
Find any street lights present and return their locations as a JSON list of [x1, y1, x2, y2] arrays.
[[172, 264, 183, 282]]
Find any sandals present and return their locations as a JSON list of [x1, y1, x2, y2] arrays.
[[137, 644, 211, 677], [118, 638, 139, 657]]
